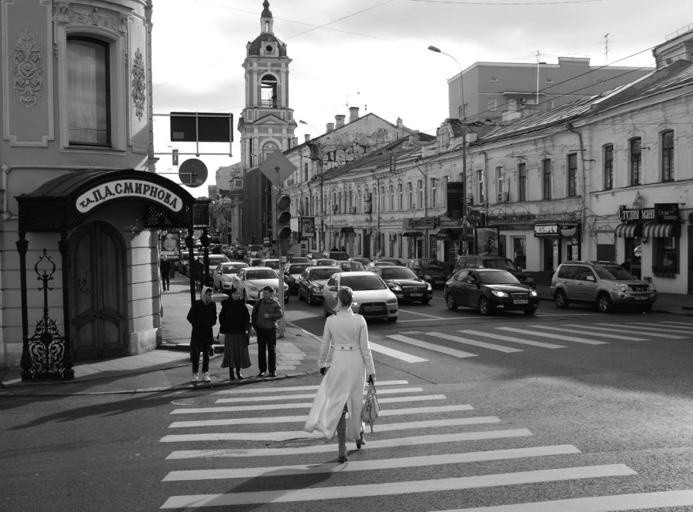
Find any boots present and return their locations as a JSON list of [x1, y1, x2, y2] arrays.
[[236, 371, 244, 379], [230, 372, 236, 380]]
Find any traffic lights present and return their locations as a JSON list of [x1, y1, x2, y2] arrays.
[[271, 192, 291, 242]]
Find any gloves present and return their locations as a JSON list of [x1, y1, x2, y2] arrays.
[[320, 367, 326, 376]]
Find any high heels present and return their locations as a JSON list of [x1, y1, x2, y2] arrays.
[[355, 432, 365, 449], [339, 456, 348, 463]]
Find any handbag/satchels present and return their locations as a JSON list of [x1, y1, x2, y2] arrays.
[[251, 299, 262, 328], [360, 376, 382, 423]]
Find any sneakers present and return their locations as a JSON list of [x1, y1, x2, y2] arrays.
[[201, 371, 212, 382], [192, 372, 200, 381], [258, 370, 266, 377], [164, 286, 170, 291], [268, 371, 277, 377]]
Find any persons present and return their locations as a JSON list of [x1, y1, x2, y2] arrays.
[[303, 285, 377, 464], [251, 286, 283, 377], [160, 254, 171, 292], [186, 286, 217, 382], [218, 288, 251, 380], [332, 246, 338, 251], [341, 247, 346, 252], [615, 256, 632, 280]]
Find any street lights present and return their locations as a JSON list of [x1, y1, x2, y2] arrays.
[[296, 117, 325, 235], [427, 44, 470, 230]]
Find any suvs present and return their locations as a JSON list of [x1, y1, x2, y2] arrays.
[[550, 259, 657, 315]]
